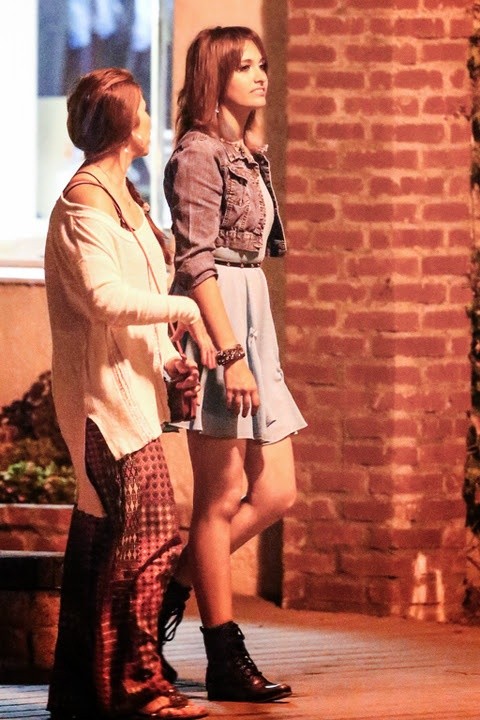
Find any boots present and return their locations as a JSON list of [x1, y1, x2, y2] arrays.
[[201, 621, 291, 703], [157, 585, 191, 682]]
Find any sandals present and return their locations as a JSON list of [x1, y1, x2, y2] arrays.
[[53, 690, 134, 720], [117, 679, 209, 720]]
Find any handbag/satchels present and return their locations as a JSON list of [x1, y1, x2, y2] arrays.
[[166, 377, 196, 422]]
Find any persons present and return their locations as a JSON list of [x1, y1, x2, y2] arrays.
[[44, 69, 217, 720], [157, 26, 308, 700]]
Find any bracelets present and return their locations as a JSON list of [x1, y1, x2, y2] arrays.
[[215, 344, 245, 366]]
